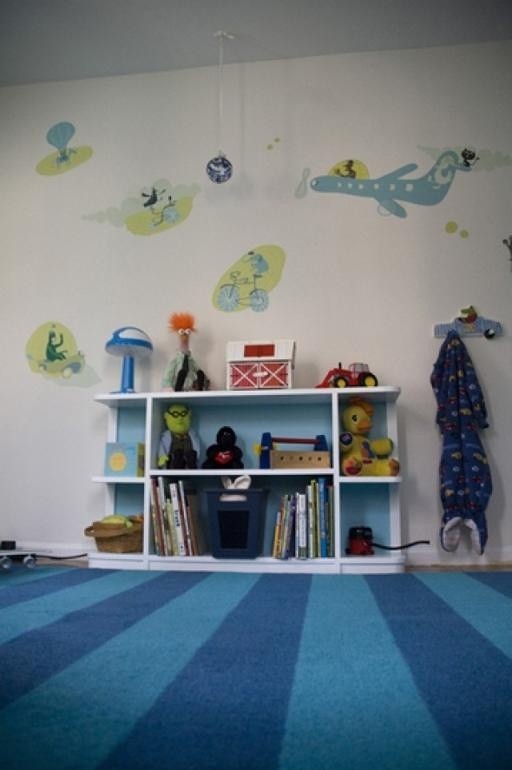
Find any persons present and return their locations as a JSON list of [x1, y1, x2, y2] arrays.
[[335, 159, 356, 179], [244, 251, 270, 275], [46, 329, 68, 362]]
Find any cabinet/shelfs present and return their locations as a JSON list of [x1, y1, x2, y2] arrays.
[[86, 386, 409, 577]]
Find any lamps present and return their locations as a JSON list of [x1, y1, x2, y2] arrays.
[[204, 29, 237, 184]]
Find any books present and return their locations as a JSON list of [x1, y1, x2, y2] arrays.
[[149, 476, 210, 557], [271, 477, 336, 560]]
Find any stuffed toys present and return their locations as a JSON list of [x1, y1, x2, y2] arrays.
[[163, 310, 213, 392], [157, 402, 202, 470], [198, 423, 245, 470], [339, 397, 400, 478]]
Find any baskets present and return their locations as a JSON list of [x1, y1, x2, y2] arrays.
[[83, 515, 144, 554]]
